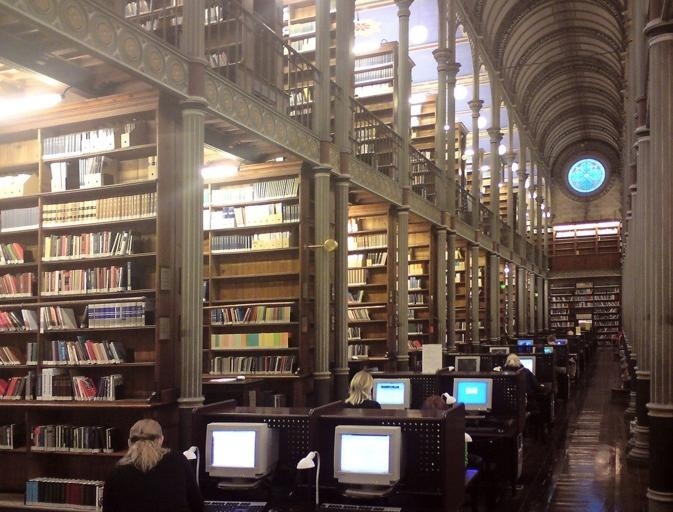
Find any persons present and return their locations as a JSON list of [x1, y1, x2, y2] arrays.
[[543, 334, 570, 397], [499, 353, 543, 412], [103, 418, 204, 512], [340, 371, 381, 408]]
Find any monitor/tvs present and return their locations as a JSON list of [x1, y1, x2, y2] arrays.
[[516, 338, 568, 353], [489, 346, 510, 354], [372, 378, 413, 408], [454, 355, 481, 372], [519, 355, 537, 376], [452, 377, 493, 418], [333, 424, 407, 497], [205, 421, 280, 490]]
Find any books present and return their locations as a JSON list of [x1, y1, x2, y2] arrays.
[[203, 176, 300, 373], [408, 247, 425, 335], [0, 335, 128, 507], [282, 7, 316, 117], [356, 128, 376, 155], [1, 229, 152, 332], [347, 216, 387, 361], [123, 0, 230, 70], [410, 150, 431, 185], [551, 283, 620, 347], [353, 53, 395, 99], [1, 122, 157, 232]]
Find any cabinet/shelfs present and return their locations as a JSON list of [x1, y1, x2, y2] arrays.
[[444, 238, 550, 356], [194, 156, 313, 506], [328, 180, 399, 378], [547, 282, 620, 354], [1, 87, 183, 509], [402, 220, 436, 361]]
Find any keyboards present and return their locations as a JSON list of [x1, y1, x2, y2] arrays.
[[320, 502, 402, 512], [204, 500, 271, 512]]
